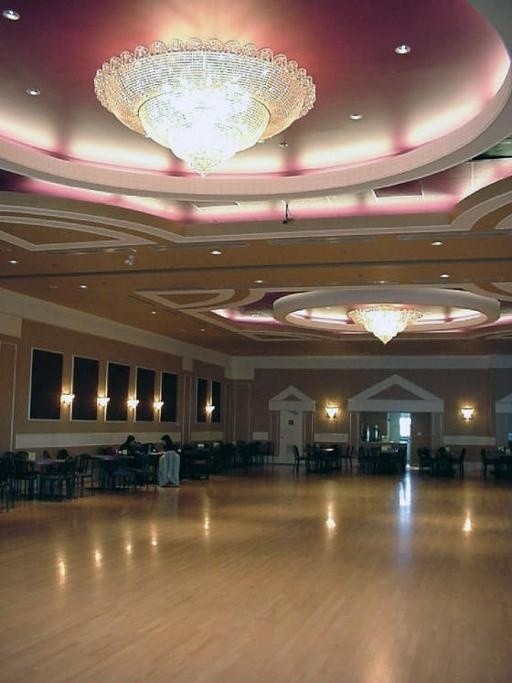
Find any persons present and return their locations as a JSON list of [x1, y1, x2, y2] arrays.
[[119, 435, 141, 456], [161, 435, 175, 452]]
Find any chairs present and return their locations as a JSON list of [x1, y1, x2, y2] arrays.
[[0, 439, 278, 515], [288, 435, 512, 481]]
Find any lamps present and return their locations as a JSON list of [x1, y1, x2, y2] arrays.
[[92, 33, 317, 175], [346, 302, 424, 345]]
